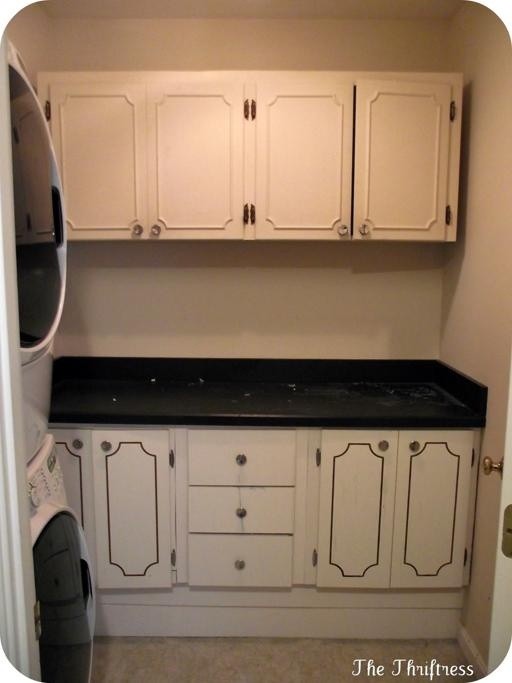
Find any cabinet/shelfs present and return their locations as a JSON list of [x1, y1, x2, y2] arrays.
[[49, 429, 170, 590], [50, 83, 244, 241], [316, 430, 475, 589], [256, 80, 454, 241], [186, 429, 295, 589]]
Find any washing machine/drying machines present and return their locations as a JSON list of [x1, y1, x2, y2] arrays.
[[7, 37, 68, 465], [25, 433, 96, 683]]
